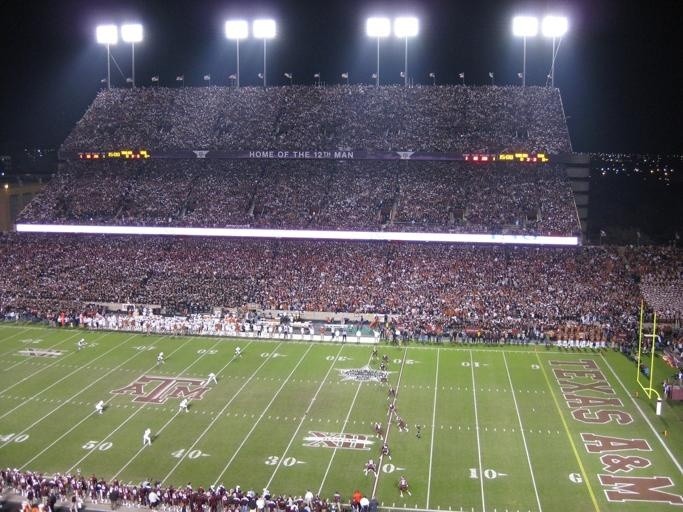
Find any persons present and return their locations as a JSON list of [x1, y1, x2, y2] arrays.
[[233, 346, 242, 359], [0, 83, 682, 349], [0, 464, 380, 512], [207, 372, 217, 384], [156, 351, 164, 365], [363, 349, 423, 497], [617, 349, 683, 403], [142, 428, 152, 446], [95, 400, 102, 413], [177, 398, 189, 413]]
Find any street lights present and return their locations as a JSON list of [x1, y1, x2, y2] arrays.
[[95, 22, 143, 89], [365, 15, 419, 88], [511, 15, 568, 89], [225, 19, 278, 88]]
